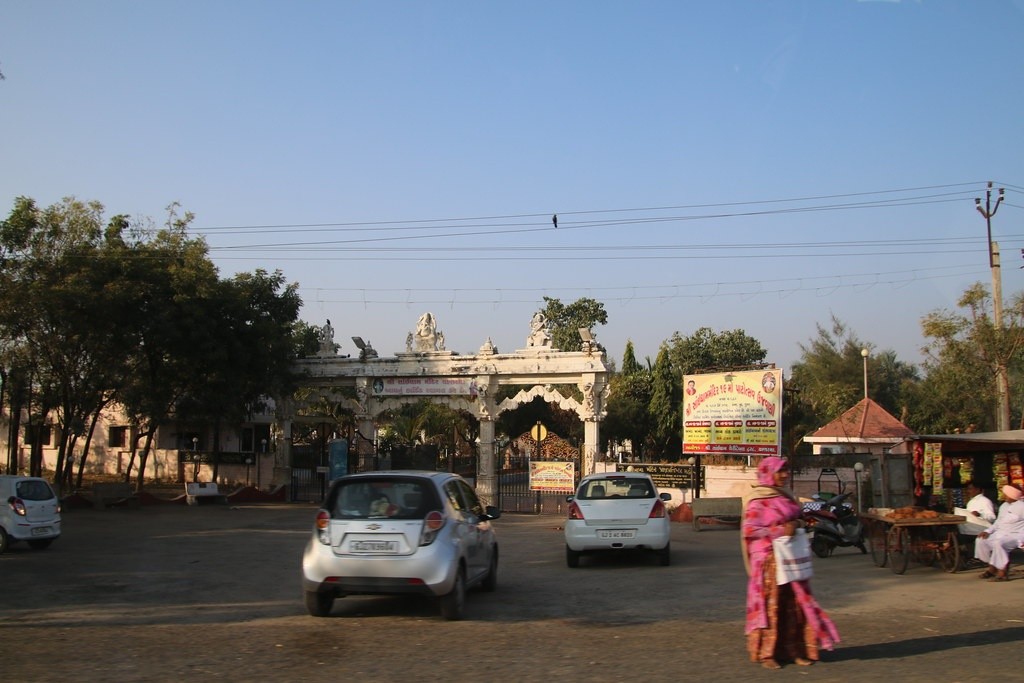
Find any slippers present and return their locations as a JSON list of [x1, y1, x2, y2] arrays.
[[979, 570, 996, 579], [988, 576, 1009, 582]]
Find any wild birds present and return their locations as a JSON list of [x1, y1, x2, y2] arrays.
[[552, 215, 558, 228]]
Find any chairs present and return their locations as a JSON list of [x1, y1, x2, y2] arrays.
[[591, 486, 606, 497], [629, 485, 646, 495]]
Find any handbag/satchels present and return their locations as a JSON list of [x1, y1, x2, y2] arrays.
[[771, 528, 813, 585]]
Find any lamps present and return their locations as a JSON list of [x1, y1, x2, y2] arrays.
[[352, 336, 367, 363]]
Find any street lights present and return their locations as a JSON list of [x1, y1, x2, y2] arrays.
[[861, 348, 869, 398]]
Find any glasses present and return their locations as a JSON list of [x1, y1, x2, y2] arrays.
[[778, 467, 786, 473]]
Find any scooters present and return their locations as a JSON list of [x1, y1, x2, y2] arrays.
[[800, 481, 867, 558]]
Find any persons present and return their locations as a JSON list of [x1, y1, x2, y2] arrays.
[[974, 483, 1024, 582], [318, 323, 341, 353], [414, 313, 438, 351], [740, 457, 841, 669], [527, 310, 552, 346], [952, 481, 996, 569]]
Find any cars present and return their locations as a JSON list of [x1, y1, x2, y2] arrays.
[[0, 473, 64, 554], [302, 469, 503, 621], [563, 472, 674, 568]]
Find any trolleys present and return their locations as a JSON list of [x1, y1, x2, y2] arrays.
[[857, 505, 967, 575]]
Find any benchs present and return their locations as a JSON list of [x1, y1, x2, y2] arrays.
[[184, 482, 227, 505], [92, 482, 140, 511], [691, 497, 742, 532]]
